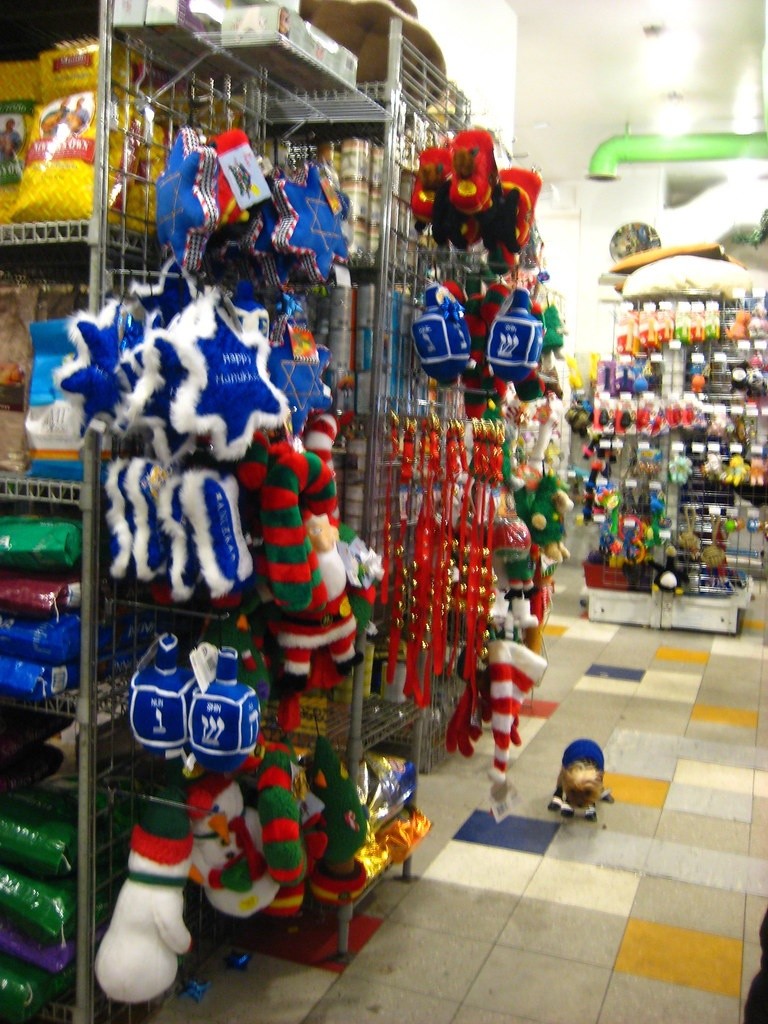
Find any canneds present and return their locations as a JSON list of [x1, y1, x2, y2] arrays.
[[310, 135, 426, 576]]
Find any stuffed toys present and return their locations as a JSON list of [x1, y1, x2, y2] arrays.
[[531, 473, 574, 563], [504, 556, 540, 600], [410, 129, 543, 265], [541, 304, 569, 368], [239, 508, 365, 700], [94, 803, 192, 1002], [189, 781, 279, 917]]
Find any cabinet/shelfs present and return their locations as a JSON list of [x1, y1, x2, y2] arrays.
[[0, 0, 767, 1024]]
